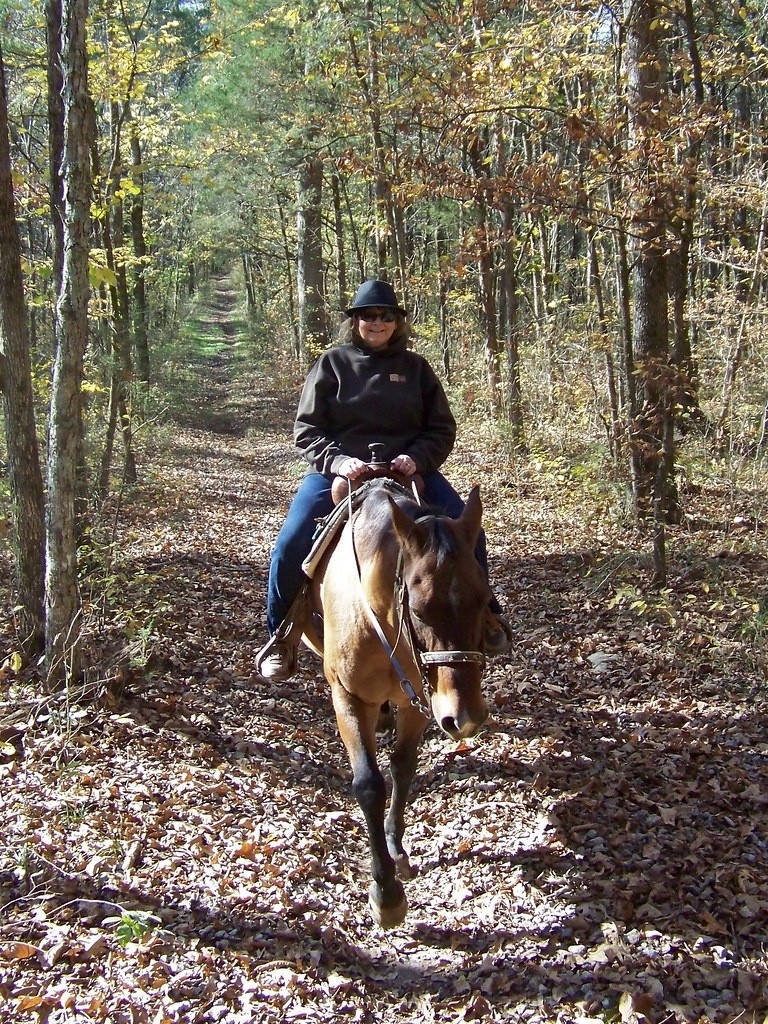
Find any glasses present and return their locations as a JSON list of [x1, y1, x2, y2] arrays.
[[358, 310, 397, 323]]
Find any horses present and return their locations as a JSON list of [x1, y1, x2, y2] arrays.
[[287, 475, 499, 931]]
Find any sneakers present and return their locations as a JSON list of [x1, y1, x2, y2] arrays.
[[261, 639, 298, 683], [483, 614, 511, 657]]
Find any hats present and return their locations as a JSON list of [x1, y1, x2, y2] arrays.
[[344, 280, 408, 318]]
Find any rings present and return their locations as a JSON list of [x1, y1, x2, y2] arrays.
[[406, 463, 411, 468]]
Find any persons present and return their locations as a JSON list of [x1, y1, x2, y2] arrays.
[[260, 281, 516, 680]]
